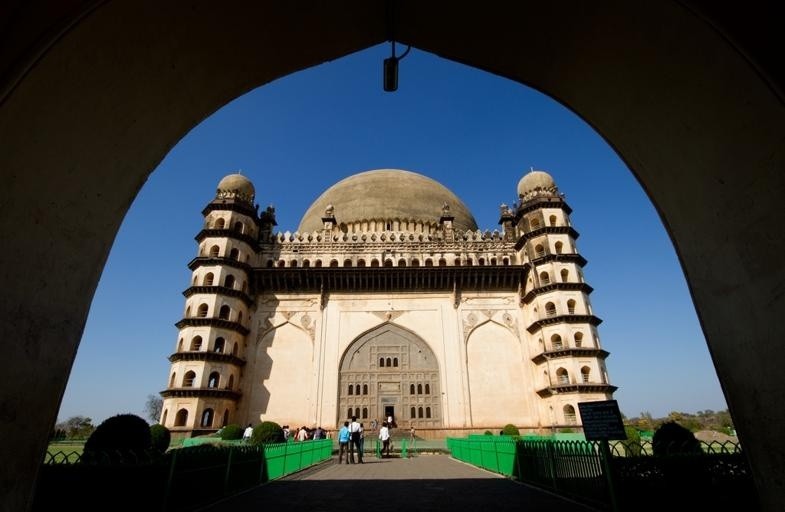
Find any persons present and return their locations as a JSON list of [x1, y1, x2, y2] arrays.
[[359, 422, 364, 457], [242, 424, 253, 441], [379, 422, 391, 457], [336, 421, 350, 464], [383, 415, 393, 428], [282, 425, 332, 441], [348, 416, 364, 464], [370, 419, 378, 432], [409, 427, 415, 441]]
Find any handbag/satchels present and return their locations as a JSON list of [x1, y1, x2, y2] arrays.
[[352, 432, 360, 441]]
